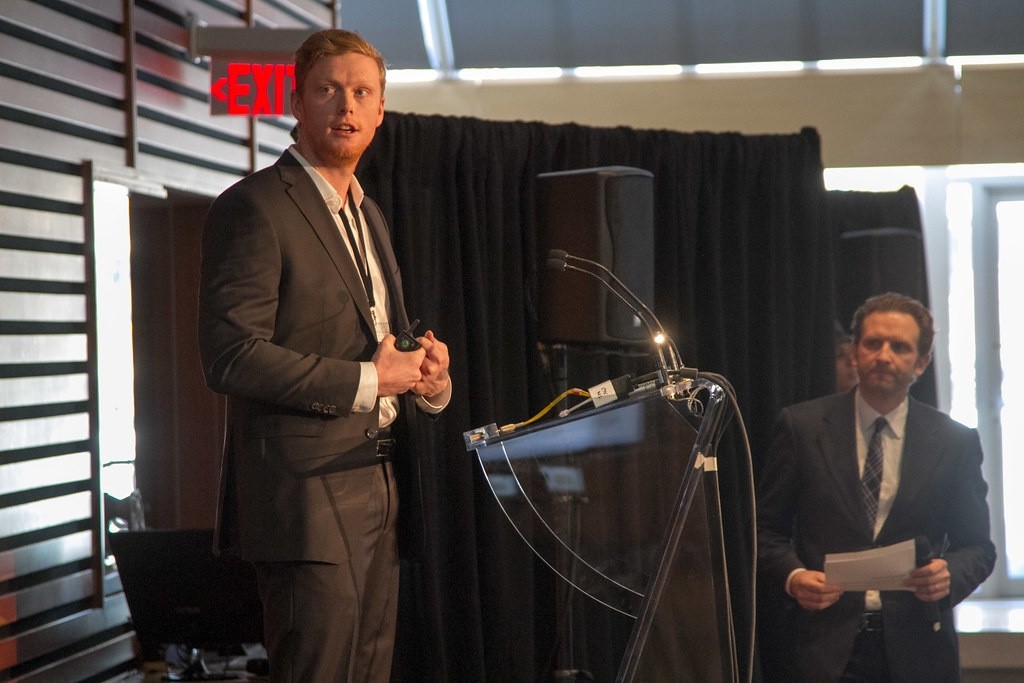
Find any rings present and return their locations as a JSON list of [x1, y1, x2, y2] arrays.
[[933, 583, 938, 593]]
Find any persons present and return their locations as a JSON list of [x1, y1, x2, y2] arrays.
[[834, 334, 859, 393], [755, 292, 998, 682], [196, 29, 452, 683]]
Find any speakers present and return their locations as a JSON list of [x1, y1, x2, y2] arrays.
[[535, 165, 656, 347]]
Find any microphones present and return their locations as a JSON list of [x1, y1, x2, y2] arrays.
[[543, 249, 686, 388]]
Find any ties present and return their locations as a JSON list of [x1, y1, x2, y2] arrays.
[[861, 417, 888, 540]]
